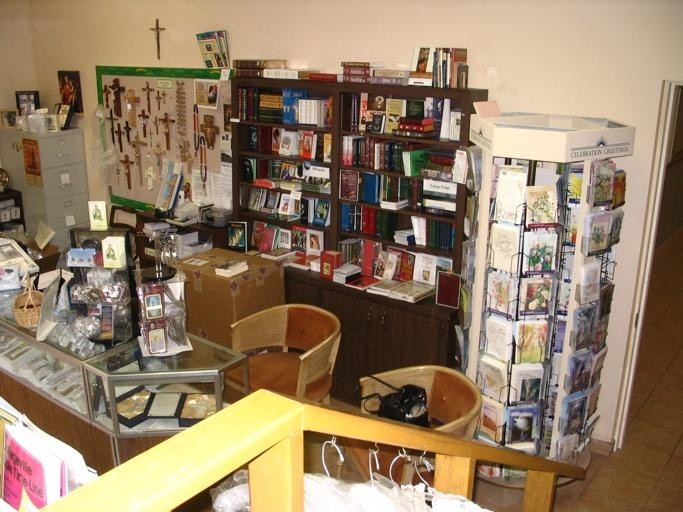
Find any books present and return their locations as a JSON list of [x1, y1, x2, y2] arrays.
[[1, 394, 100, 512], [228, 47, 469, 304], [155, 173, 183, 210], [478, 158, 626, 479], [214, 260, 249, 277]]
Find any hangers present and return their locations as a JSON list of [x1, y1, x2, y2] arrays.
[[317, 440, 438, 489]]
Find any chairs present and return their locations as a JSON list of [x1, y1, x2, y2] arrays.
[[337, 364, 483, 487], [225, 304, 342, 411]]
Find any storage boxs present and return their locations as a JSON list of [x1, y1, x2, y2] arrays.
[[171, 248, 286, 351]]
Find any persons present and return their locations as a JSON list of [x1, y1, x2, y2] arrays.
[[63, 76, 73, 103], [67, 80, 78, 104]]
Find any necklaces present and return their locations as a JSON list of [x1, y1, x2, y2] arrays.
[[192, 103, 200, 156], [198, 136, 208, 182]]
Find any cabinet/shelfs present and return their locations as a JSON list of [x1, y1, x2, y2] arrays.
[[228, 75, 477, 406], [1, 128, 88, 269], [0, 289, 249, 476]]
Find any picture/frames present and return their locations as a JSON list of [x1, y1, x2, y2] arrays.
[[15, 90, 40, 115]]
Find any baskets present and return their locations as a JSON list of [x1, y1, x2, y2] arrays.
[[12, 270, 43, 328]]
